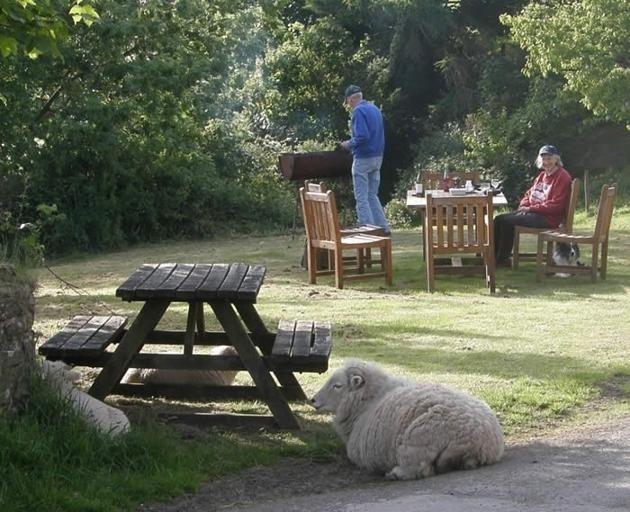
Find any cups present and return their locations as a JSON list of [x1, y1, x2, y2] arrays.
[[491, 179, 499, 188]]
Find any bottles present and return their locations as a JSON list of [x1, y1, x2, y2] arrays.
[[443, 164, 449, 192], [416, 169, 425, 197], [466, 181, 472, 191]]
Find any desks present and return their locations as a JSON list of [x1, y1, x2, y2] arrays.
[[86, 259, 302, 431]]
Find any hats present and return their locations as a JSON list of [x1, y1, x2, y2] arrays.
[[345, 85, 362, 103], [538, 145, 559, 156]]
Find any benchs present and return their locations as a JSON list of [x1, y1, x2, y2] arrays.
[[34, 314, 330, 431]]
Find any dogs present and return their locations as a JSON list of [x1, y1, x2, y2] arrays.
[[551, 241, 588, 279]]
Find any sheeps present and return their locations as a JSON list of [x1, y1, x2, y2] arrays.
[[308, 354, 507, 482], [39, 358, 133, 440], [125, 344, 242, 387]]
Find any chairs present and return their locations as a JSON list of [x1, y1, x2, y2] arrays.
[[512, 178, 621, 285], [422, 171, 494, 292], [298, 178, 392, 290]]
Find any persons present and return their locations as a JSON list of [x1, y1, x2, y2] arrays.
[[337, 85, 393, 238], [493, 144, 571, 268]]
